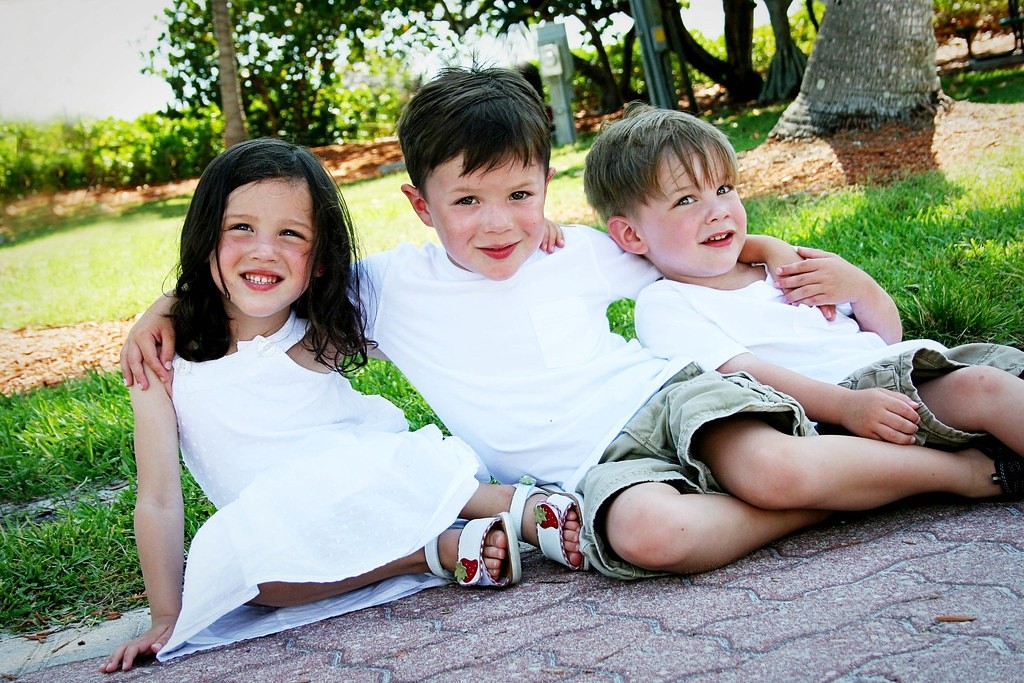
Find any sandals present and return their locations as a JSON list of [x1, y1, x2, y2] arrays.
[[507, 482, 592, 572], [423, 510, 522, 587]]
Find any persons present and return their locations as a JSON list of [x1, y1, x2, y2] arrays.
[[100, 138, 588, 671], [583, 101, 1024, 446], [122, 66, 1024, 575]]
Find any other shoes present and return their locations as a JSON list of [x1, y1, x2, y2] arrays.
[[965, 433, 1024, 500]]
